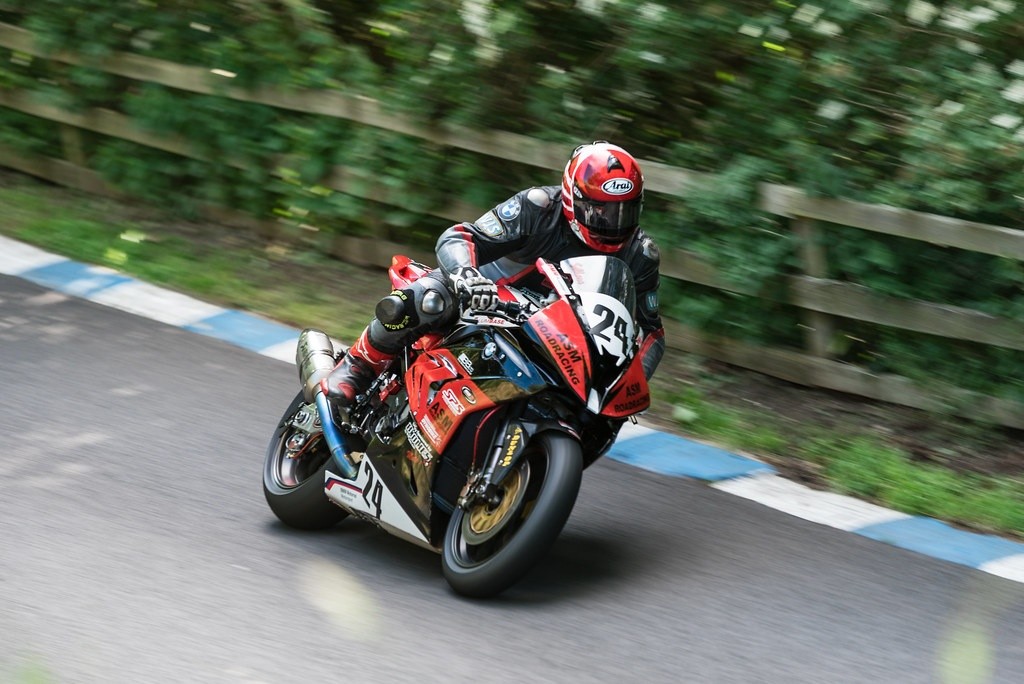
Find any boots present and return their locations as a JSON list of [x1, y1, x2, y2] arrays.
[[320, 323, 401, 406]]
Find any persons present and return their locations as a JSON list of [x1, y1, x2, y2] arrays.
[[321, 140, 666, 470]]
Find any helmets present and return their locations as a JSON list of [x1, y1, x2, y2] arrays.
[[562, 139, 644, 253]]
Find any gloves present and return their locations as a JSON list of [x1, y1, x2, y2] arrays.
[[447, 267, 499, 319]]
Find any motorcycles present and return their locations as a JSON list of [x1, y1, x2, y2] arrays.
[[260, 253, 654, 598]]
